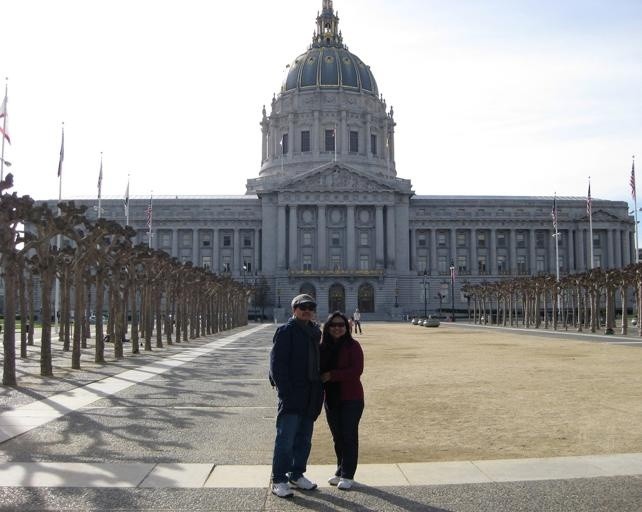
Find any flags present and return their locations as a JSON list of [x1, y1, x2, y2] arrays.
[[1, 80, 14, 170], [628, 159, 636, 201], [54, 124, 66, 177], [122, 179, 131, 217], [585, 180, 592, 216], [145, 195, 152, 229], [551, 194, 559, 229], [96, 154, 104, 199]]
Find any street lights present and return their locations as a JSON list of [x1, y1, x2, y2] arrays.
[[422, 271, 430, 318]]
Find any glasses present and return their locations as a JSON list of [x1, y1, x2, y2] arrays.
[[294, 305, 316, 312], [328, 321, 346, 328]]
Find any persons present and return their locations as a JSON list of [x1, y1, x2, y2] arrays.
[[353, 308, 364, 335], [319, 311, 365, 490], [267, 292, 323, 497], [348, 317, 354, 333]]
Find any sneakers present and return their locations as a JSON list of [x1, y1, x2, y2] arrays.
[[270, 476, 317, 500], [328, 475, 355, 490]]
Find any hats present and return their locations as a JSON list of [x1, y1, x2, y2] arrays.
[[289, 293, 318, 311]]
